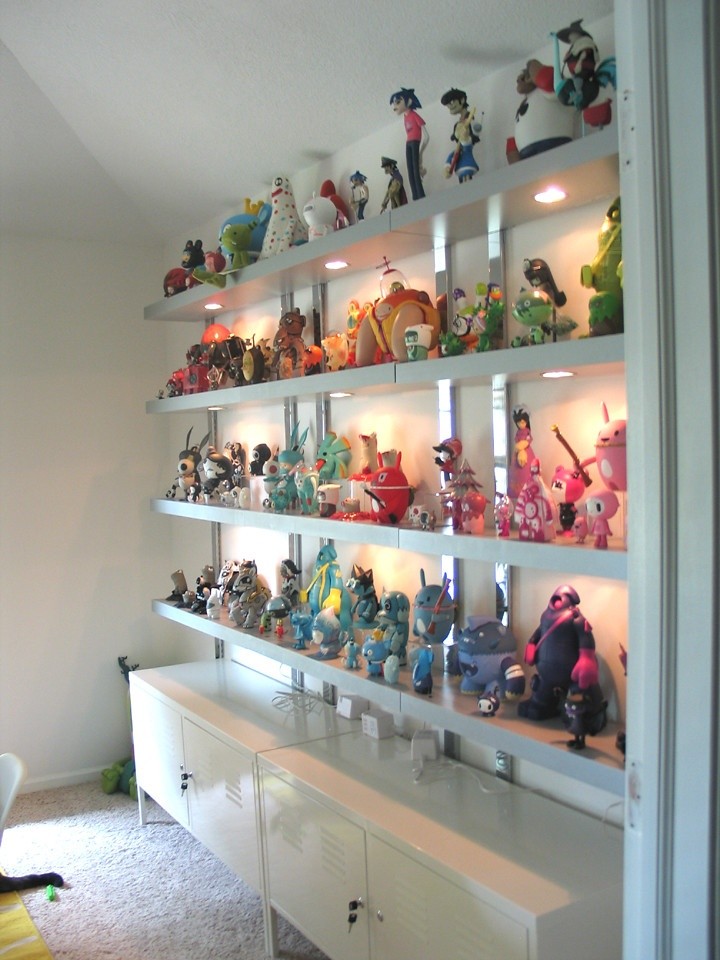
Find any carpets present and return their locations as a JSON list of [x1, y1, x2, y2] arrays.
[[0, 868, 54, 960]]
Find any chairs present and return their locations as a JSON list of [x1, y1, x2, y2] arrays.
[[0, 753, 27, 847]]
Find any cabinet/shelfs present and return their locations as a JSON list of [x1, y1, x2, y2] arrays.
[[128, 659, 624, 960], [141, 122, 627, 796]]
